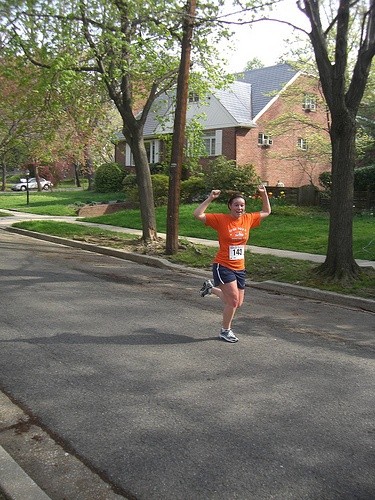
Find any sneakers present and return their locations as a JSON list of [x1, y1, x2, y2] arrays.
[[218, 328, 239, 343], [199, 280, 213, 298]]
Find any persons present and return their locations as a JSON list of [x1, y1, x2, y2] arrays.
[[192, 185, 272, 342]]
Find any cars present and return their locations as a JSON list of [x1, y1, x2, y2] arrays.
[[11, 178, 53, 191]]
